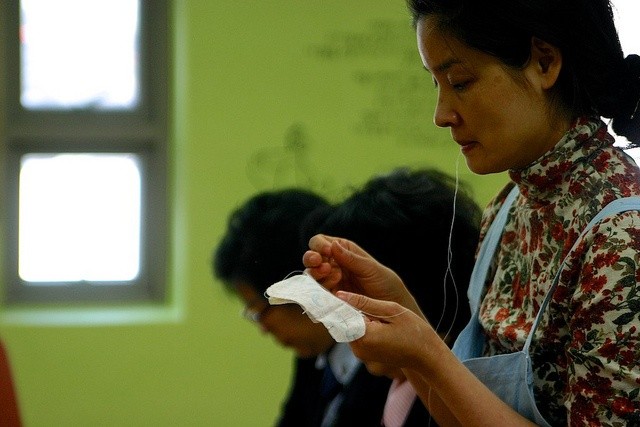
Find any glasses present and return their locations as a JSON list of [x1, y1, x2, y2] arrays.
[[241, 303, 274, 325]]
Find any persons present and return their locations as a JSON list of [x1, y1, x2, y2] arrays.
[[298, 0, 640, 427], [210, 184, 365, 427], [314, 162, 484, 427]]
